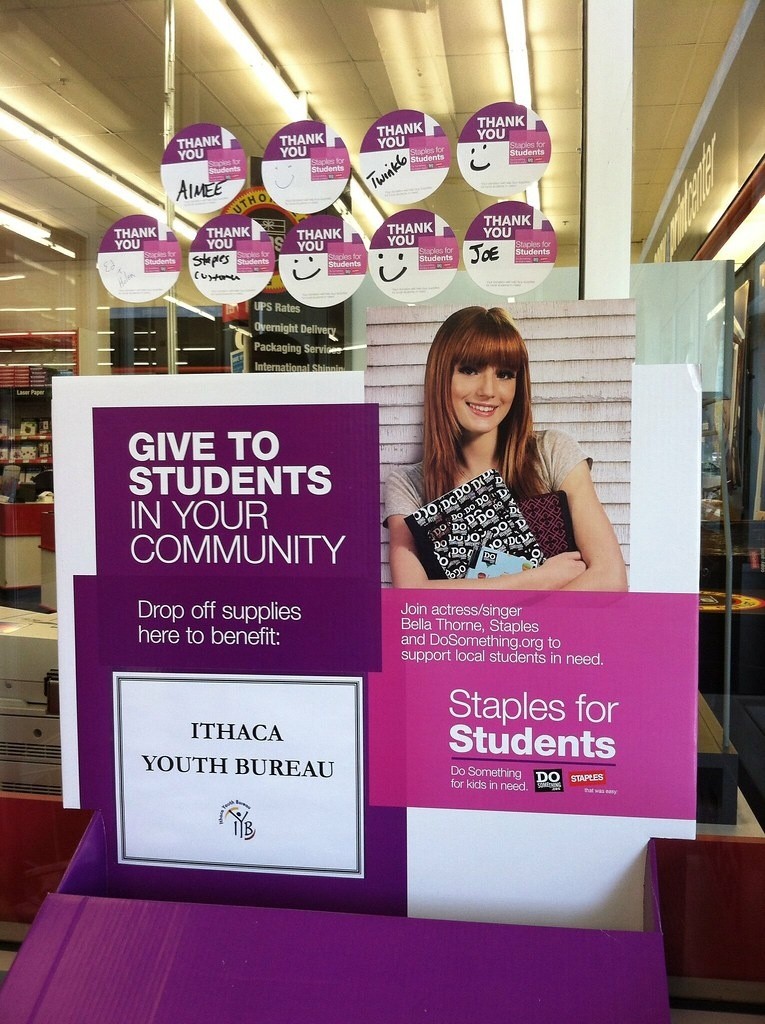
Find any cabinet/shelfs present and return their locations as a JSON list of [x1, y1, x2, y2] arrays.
[[0, 329, 79, 463]]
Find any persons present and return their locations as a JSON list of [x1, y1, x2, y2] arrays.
[[382, 305, 628, 593]]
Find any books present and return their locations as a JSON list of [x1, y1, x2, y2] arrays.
[[403, 468, 577, 580]]
